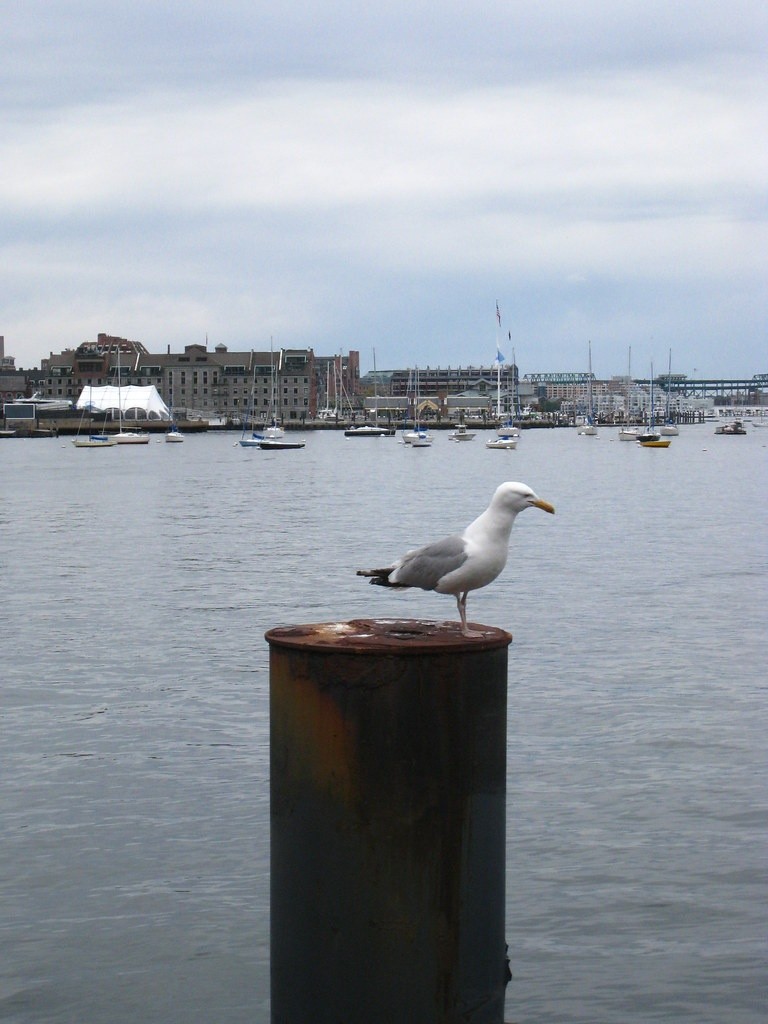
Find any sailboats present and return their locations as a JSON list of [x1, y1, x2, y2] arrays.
[[257, 360, 306, 450], [89, 347, 150, 444], [71, 365, 118, 447], [318, 346, 437, 446], [497, 348, 521, 438], [636, 361, 661, 441], [485, 376, 518, 449], [238, 335, 286, 446], [164, 391, 185, 443], [659, 347, 679, 436], [577, 340, 598, 436], [618, 345, 643, 441]]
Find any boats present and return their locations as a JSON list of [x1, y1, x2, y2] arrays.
[[714, 417, 768, 435], [638, 440, 672, 447], [448, 424, 477, 441]]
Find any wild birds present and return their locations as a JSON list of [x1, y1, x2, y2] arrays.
[[355, 481, 556, 640]]
